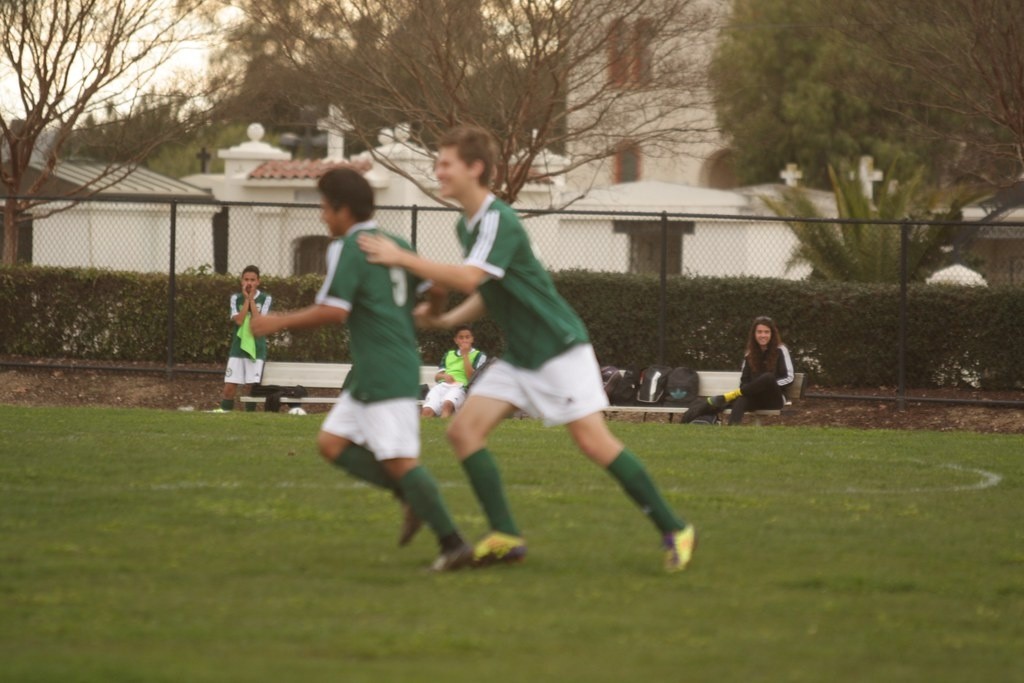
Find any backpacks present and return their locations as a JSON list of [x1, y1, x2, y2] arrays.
[[613, 367, 641, 406], [680, 401, 721, 425], [663, 366, 698, 407], [599, 365, 621, 400], [633, 365, 673, 407]]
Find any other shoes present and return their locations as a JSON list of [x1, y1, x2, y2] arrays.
[[708, 395, 728, 408], [397, 505, 422, 547], [431, 541, 475, 573]]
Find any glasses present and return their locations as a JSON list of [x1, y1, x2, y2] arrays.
[[755, 316, 771, 322]]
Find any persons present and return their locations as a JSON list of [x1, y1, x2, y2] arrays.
[[420, 325, 488, 419], [213, 264, 272, 411], [248, 165, 470, 573], [356, 122, 696, 576], [707, 316, 794, 426]]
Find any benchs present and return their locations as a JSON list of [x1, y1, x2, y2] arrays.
[[240, 362, 438, 413], [605, 370, 805, 422]]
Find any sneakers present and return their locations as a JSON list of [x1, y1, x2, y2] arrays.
[[466, 532, 525, 565], [660, 524, 698, 574]]
